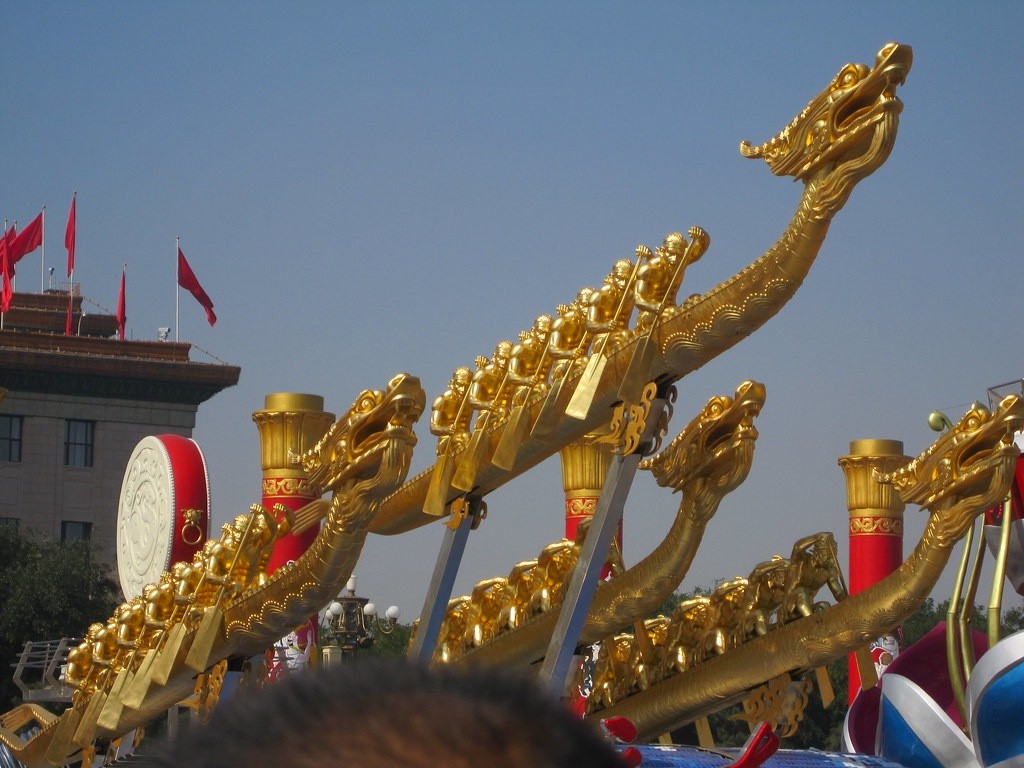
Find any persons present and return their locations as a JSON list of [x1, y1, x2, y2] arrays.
[[563, 531, 844, 722], [409, 516, 619, 674], [430, 223, 710, 461], [128, 651, 620, 768], [66, 504, 296, 699]]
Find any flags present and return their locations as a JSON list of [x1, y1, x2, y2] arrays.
[[61, 199, 75, 277], [117, 272, 127, 338], [176, 246, 217, 329], [0, 205, 45, 312]]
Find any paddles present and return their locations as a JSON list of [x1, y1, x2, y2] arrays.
[[411, 516, 880, 750], [422, 231, 699, 518], [42, 510, 257, 768]]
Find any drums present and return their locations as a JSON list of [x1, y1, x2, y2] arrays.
[[117, 433, 213, 604]]
